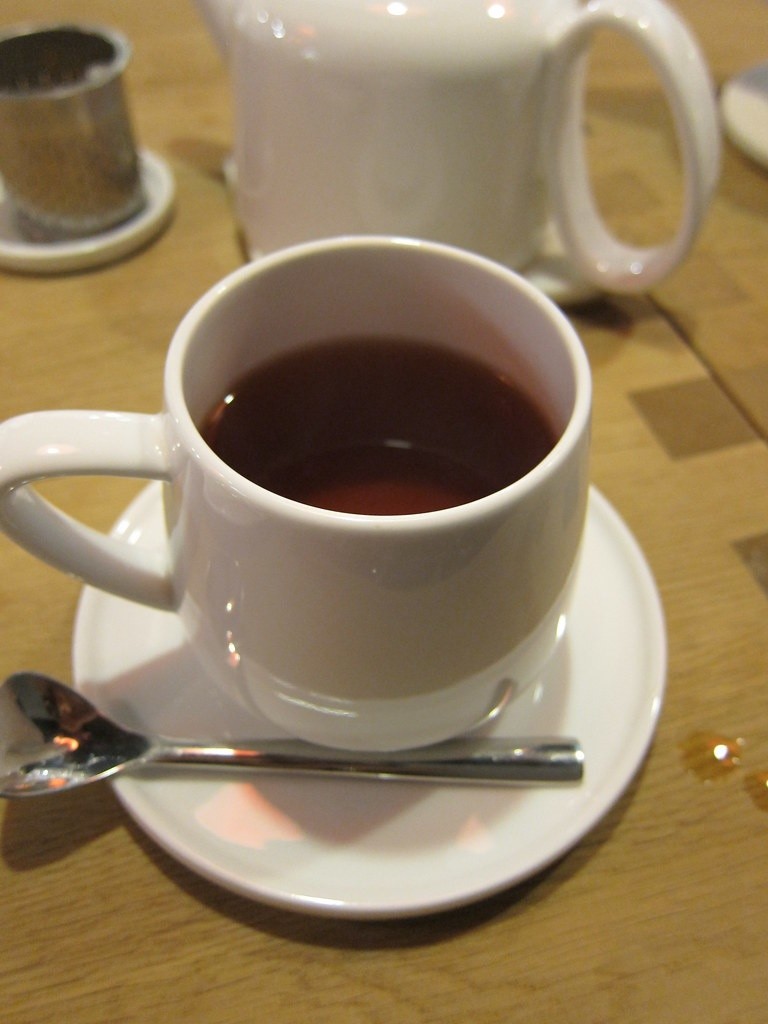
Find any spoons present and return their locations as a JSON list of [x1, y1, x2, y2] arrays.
[[1, 672, 583, 801]]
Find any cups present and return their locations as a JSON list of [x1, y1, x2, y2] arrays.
[[0, 24, 146, 236]]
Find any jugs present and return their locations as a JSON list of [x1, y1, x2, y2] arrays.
[[192, 0, 719, 305]]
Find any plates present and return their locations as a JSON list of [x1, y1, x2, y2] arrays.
[[720, 65, 767, 168], [1, 152, 176, 272], [1, 234, 595, 751], [72, 475, 664, 918]]
